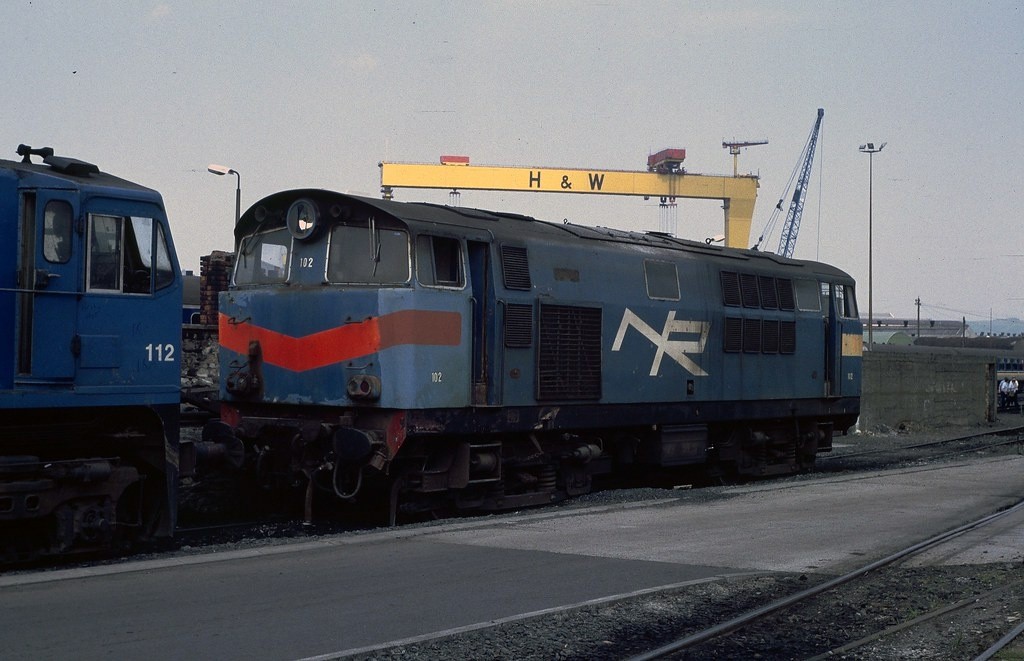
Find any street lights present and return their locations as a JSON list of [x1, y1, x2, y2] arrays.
[[206, 163, 242, 284], [858, 141, 887, 350]]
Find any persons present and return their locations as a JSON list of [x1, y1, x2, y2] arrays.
[[998, 377, 1019, 409]]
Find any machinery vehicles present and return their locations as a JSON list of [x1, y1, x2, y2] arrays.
[[750, 109, 824, 261]]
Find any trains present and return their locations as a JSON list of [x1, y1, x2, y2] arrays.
[[0, 145, 863, 560]]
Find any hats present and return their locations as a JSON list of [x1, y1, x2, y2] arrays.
[[1012, 376, 1015, 380]]
